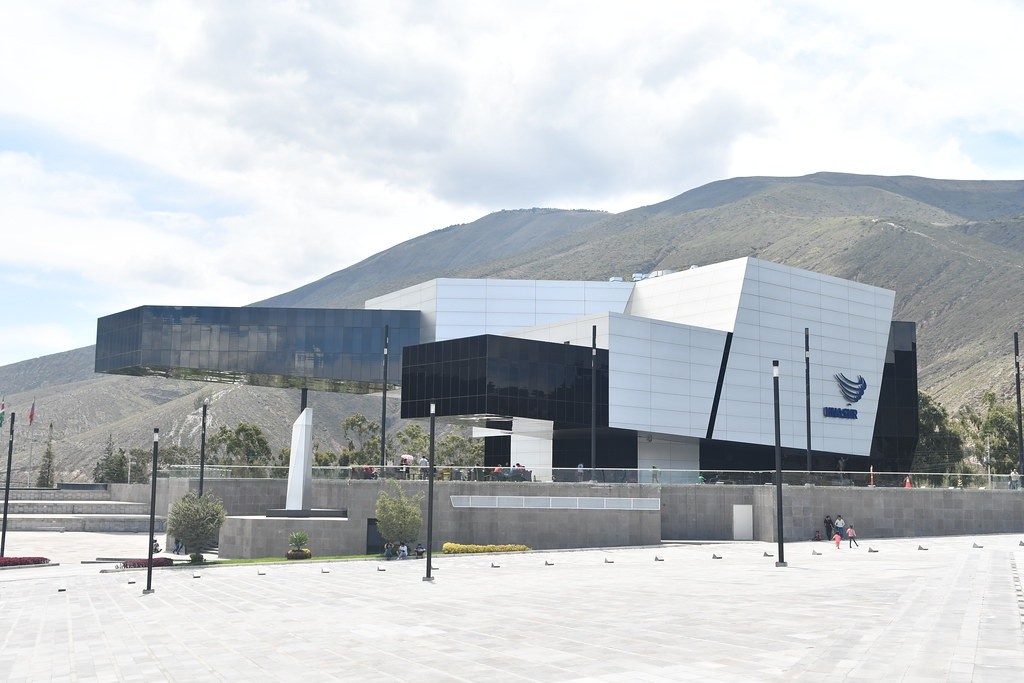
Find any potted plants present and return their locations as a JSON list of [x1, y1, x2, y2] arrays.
[[286, 532, 312, 560]]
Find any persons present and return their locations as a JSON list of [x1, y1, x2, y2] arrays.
[[846, 525, 858, 548], [652, 464, 661, 482], [1008, 468, 1020, 489], [400, 457, 409, 473], [450, 463, 467, 481], [577, 464, 584, 482], [467, 463, 480, 481], [832, 531, 841, 549], [384, 542, 395, 560], [836, 456, 849, 476], [172, 538, 183, 555], [415, 544, 425, 559], [698, 474, 707, 484], [494, 462, 525, 481], [396, 542, 408, 559], [835, 515, 845, 539], [419, 455, 436, 479], [824, 515, 835, 541]]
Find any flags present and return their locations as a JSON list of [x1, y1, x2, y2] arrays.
[[28, 403, 34, 425], [0, 400, 5, 426]]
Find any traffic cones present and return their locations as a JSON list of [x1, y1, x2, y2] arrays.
[[905, 474, 912, 487]]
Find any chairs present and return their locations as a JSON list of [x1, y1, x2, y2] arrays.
[[350, 467, 533, 482]]
